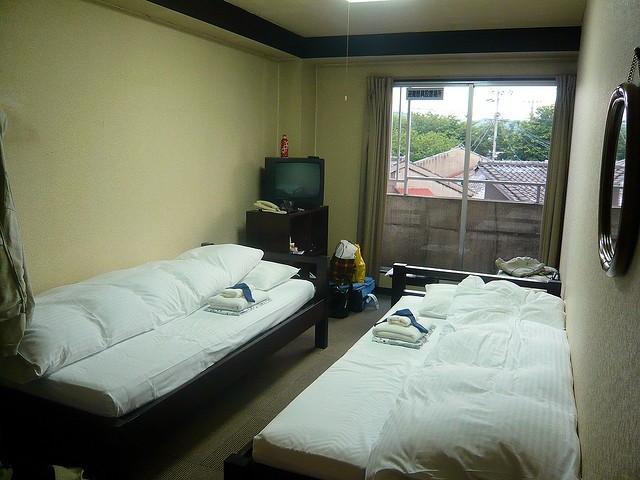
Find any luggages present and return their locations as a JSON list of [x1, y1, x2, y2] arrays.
[[328, 277, 376, 313]]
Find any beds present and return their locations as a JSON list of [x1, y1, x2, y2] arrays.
[[1, 243, 331, 462], [224, 262, 582, 479]]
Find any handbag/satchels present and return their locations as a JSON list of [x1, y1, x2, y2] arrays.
[[351, 243, 366, 284], [325, 274, 353, 318], [331, 240, 358, 282], [335, 239, 358, 260]]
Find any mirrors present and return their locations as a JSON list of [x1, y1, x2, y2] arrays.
[[598, 82, 639, 278]]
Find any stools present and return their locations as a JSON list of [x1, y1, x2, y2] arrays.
[[497, 269, 559, 291], [384, 266, 439, 288]]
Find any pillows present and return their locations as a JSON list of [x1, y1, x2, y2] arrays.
[[239, 260, 301, 291], [417, 284, 458, 318]]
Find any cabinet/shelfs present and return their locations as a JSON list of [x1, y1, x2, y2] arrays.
[[246, 205, 328, 278]]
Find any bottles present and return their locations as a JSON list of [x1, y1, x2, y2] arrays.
[[280, 134, 289, 158]]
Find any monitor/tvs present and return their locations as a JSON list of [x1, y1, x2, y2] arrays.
[[265, 157, 324, 209]]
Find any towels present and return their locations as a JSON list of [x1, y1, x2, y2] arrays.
[[372, 321, 431, 342], [205, 299, 272, 316], [221, 284, 254, 297], [374, 326, 436, 349], [387, 312, 420, 327], [208, 291, 268, 311]]
